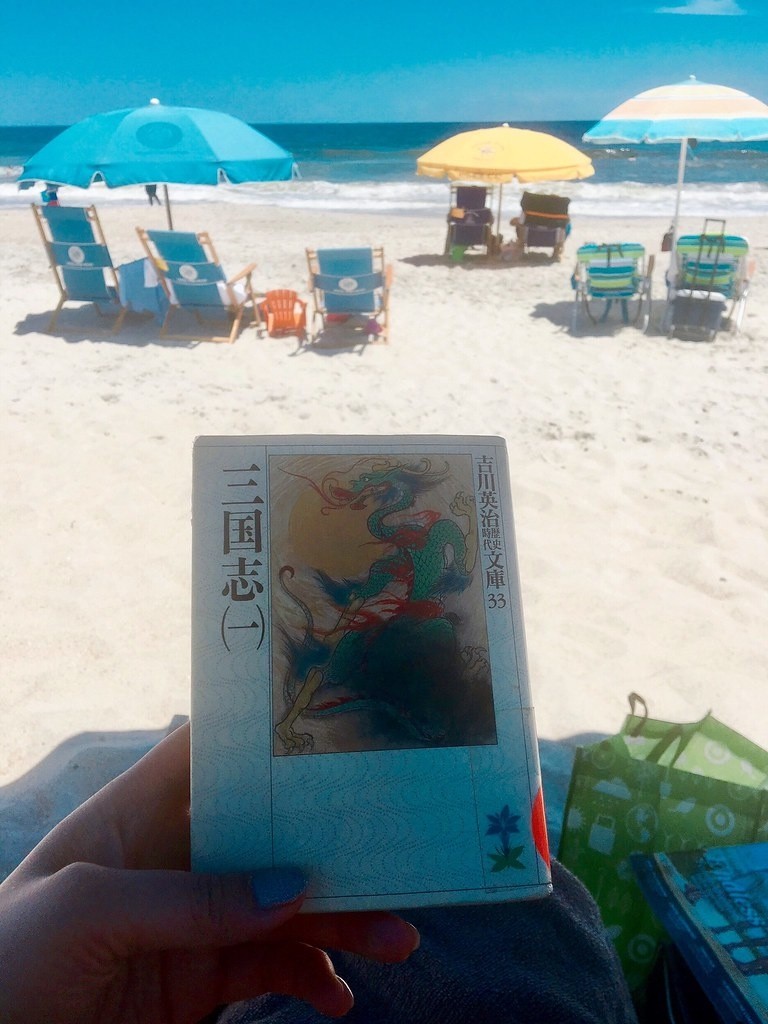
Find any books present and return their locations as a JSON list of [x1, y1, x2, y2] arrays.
[[190, 433, 553, 914]]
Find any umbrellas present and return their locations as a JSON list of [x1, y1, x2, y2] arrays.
[[416, 123, 595, 253], [582, 75, 768, 251], [16, 98, 302, 231]]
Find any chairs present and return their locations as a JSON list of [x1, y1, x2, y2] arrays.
[[658, 234, 755, 341], [443, 184, 503, 261], [570, 242, 655, 337], [30, 202, 167, 337], [509, 191, 572, 262], [304, 246, 394, 348], [135, 226, 262, 345]]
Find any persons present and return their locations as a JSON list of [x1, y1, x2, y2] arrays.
[[45, 182, 60, 206], [146, 184, 162, 207], [0, 718, 637, 1024]]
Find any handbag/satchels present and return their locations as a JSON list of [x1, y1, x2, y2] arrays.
[[556, 692, 768, 992]]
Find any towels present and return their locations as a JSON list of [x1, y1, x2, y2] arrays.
[[213, 851, 640, 1024]]
